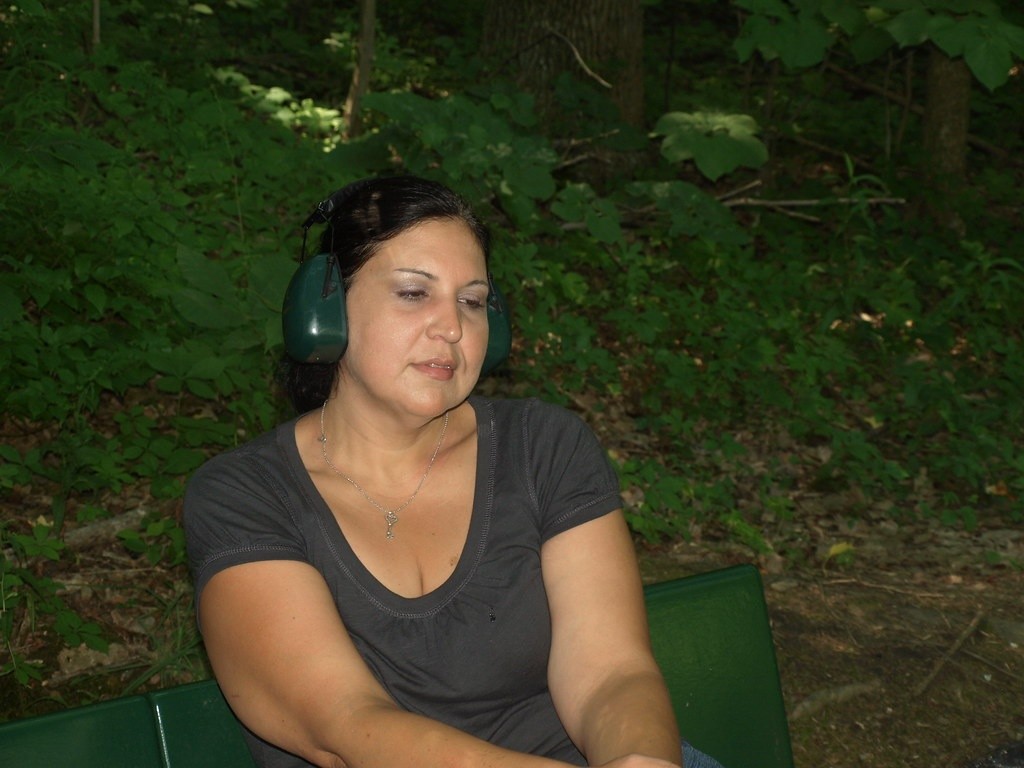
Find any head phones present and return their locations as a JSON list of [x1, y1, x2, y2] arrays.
[[284, 180, 511, 376]]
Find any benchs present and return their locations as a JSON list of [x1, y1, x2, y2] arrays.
[[1, 564, 798, 768]]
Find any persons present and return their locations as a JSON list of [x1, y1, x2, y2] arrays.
[[183, 179, 726, 768]]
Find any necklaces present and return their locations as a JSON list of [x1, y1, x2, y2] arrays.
[[318, 398, 449, 541]]
